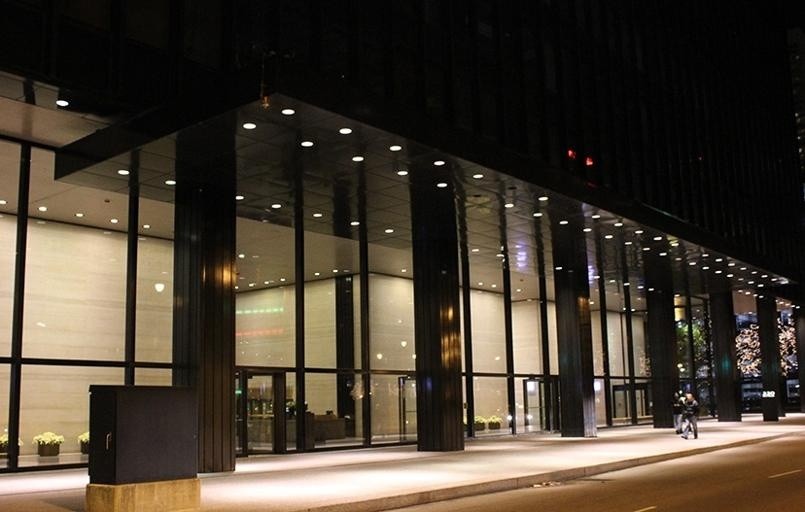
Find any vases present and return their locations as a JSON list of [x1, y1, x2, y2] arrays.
[[474, 423, 485, 431], [0, 444, 20, 459], [488, 423, 500, 429], [81, 440, 90, 454], [38, 442, 59, 457], [463, 424, 467, 431]]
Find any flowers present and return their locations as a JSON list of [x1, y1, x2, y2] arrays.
[[463, 415, 467, 425], [32, 432, 65, 446], [0, 429, 24, 448], [487, 415, 503, 424], [474, 416, 487, 424], [78, 431, 89, 444]]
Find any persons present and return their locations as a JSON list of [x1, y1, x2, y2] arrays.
[[682, 393, 700, 439], [674, 391, 683, 435]]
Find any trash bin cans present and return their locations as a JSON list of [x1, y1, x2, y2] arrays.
[[304, 411, 315, 453], [761, 387, 779, 421]]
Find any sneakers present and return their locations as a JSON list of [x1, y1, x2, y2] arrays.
[[676, 428, 698, 439]]
